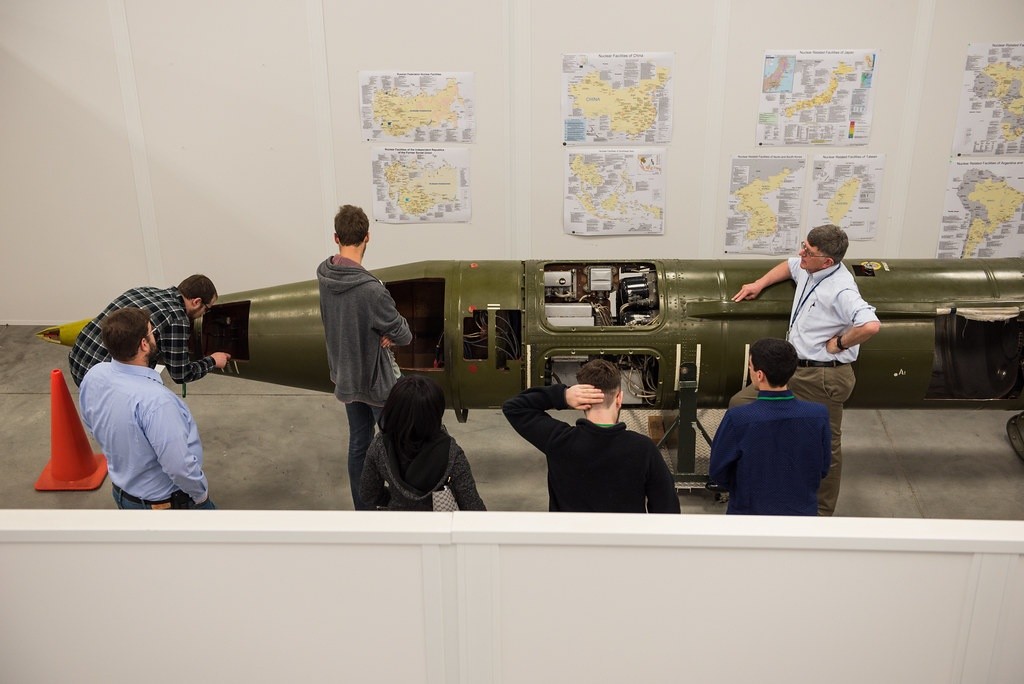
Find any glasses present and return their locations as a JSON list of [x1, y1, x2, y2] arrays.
[[801, 241, 830, 258], [204, 304, 211, 313]]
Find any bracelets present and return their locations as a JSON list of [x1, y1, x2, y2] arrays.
[[836, 335, 848, 350]]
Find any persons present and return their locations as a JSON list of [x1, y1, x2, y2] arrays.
[[355, 375, 488, 510], [317, 204, 413, 510], [705, 223, 882, 516], [502, 360, 680, 513], [68, 273, 231, 510]]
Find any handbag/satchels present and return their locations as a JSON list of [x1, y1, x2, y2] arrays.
[[432, 477, 460, 512]]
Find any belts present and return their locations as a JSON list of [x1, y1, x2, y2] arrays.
[[112, 483, 171, 504], [797, 359, 844, 367]]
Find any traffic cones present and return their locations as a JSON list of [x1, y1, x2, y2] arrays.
[[35, 369, 108, 491]]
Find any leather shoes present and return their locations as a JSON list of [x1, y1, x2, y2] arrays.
[[705, 482, 727, 492]]
